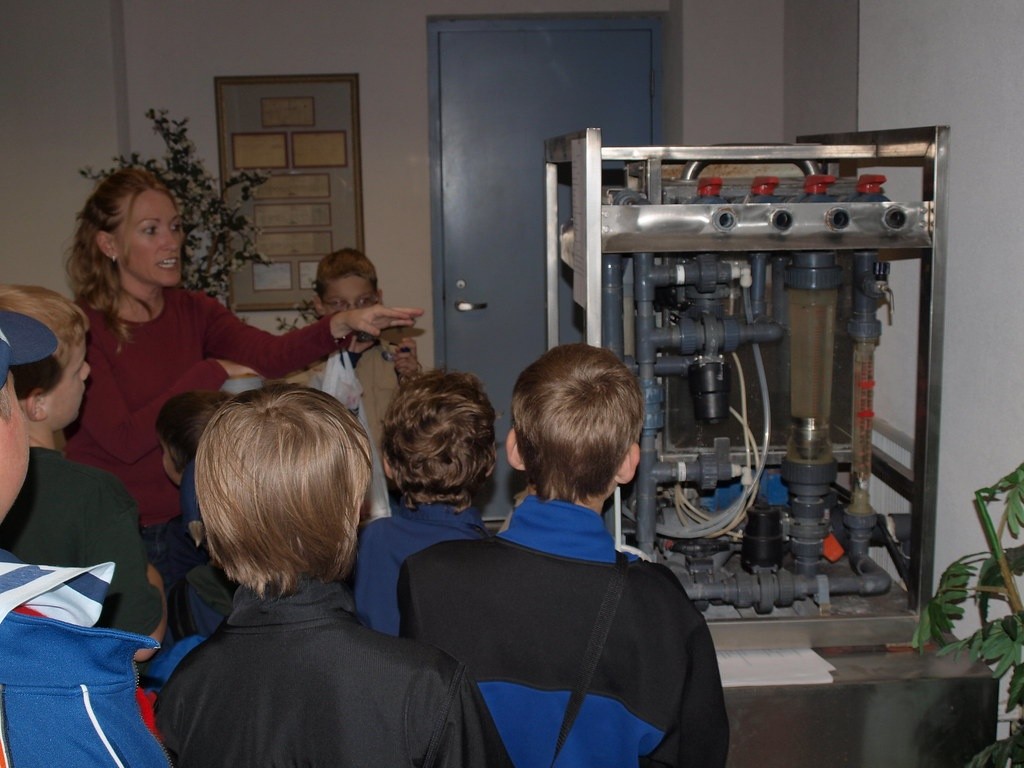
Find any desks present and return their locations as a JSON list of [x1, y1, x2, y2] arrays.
[[714, 632, 1000, 768]]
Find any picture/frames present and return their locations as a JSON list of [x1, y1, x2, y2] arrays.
[[215, 73, 365, 313]]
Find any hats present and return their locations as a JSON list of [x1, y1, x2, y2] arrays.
[[0, 309, 58, 389]]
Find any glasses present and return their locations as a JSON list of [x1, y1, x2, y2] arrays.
[[321, 294, 378, 312]]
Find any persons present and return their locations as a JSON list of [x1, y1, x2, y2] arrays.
[[0, 171, 731, 768]]
